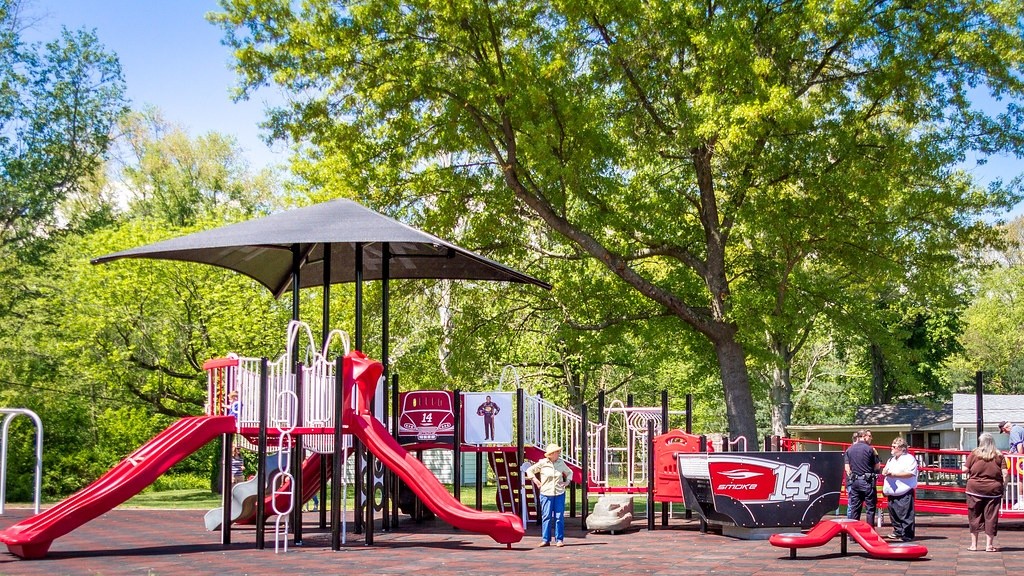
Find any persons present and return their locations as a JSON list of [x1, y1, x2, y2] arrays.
[[962, 433, 1008, 551], [222, 391, 243, 423], [527, 443, 573, 547], [232, 445, 244, 486], [476, 396, 500, 441], [882, 437, 918, 542], [998, 420, 1024, 509], [845, 429, 883, 544]]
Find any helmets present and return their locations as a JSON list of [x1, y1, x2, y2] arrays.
[[478, 410, 484, 416]]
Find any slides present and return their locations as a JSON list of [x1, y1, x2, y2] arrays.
[[203, 447, 355, 532], [348, 412, 525, 543], [0, 416, 236, 544]]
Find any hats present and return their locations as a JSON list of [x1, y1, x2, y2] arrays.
[[544, 444, 562, 458], [998, 421, 1008, 434]]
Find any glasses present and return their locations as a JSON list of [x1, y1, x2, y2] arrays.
[[891, 446, 899, 448], [865, 435, 871, 438]]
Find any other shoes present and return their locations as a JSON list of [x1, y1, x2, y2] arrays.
[[985, 547, 997, 552], [849, 537, 855, 543], [967, 547, 977, 551], [888, 534, 903, 542], [556, 541, 563, 547], [1013, 502, 1024, 510], [539, 542, 550, 547]]
